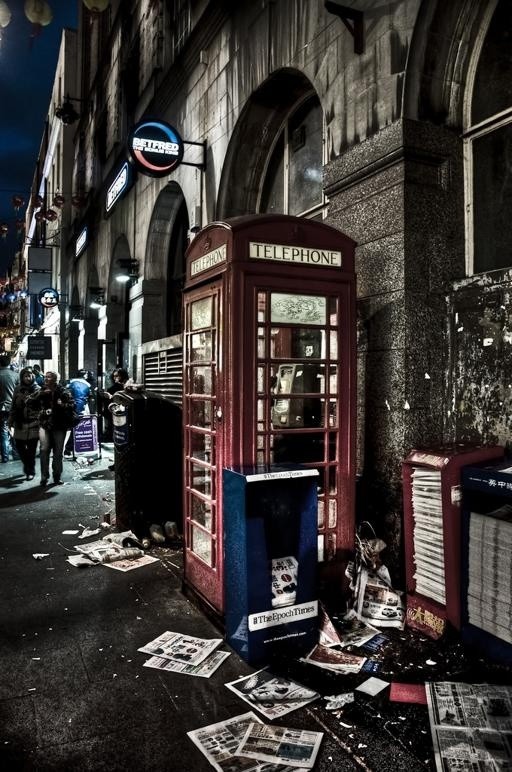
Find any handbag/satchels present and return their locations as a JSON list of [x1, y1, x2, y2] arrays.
[[62, 407, 81, 430]]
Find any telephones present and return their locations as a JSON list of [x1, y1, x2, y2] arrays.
[[270, 363, 321, 427]]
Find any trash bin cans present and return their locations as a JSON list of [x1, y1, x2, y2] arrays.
[[112, 389, 183, 540]]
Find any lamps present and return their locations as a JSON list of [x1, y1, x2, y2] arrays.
[[54, 92, 82, 126], [69, 305, 84, 322], [0, 189, 88, 236], [88, 287, 104, 309], [115, 259, 139, 286]]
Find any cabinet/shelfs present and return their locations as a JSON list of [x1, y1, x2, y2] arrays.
[[460, 455, 512, 686], [223, 462, 321, 671], [401, 443, 505, 642]]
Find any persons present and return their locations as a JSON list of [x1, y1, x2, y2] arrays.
[[361, 584, 406, 623], [8, 367, 43, 481], [98, 367, 130, 472], [0, 354, 22, 463], [25, 371, 78, 486], [8, 363, 103, 462], [240, 667, 295, 709]]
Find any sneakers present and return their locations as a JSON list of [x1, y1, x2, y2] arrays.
[[40, 479, 64, 487], [27, 474, 34, 481]]
